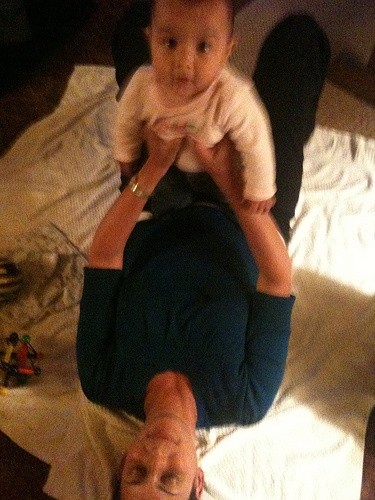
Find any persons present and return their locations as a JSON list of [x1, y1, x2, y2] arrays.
[[113, 0, 278, 222], [76, 0, 332, 500]]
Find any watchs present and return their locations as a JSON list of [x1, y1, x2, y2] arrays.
[[127, 174, 150, 199]]
